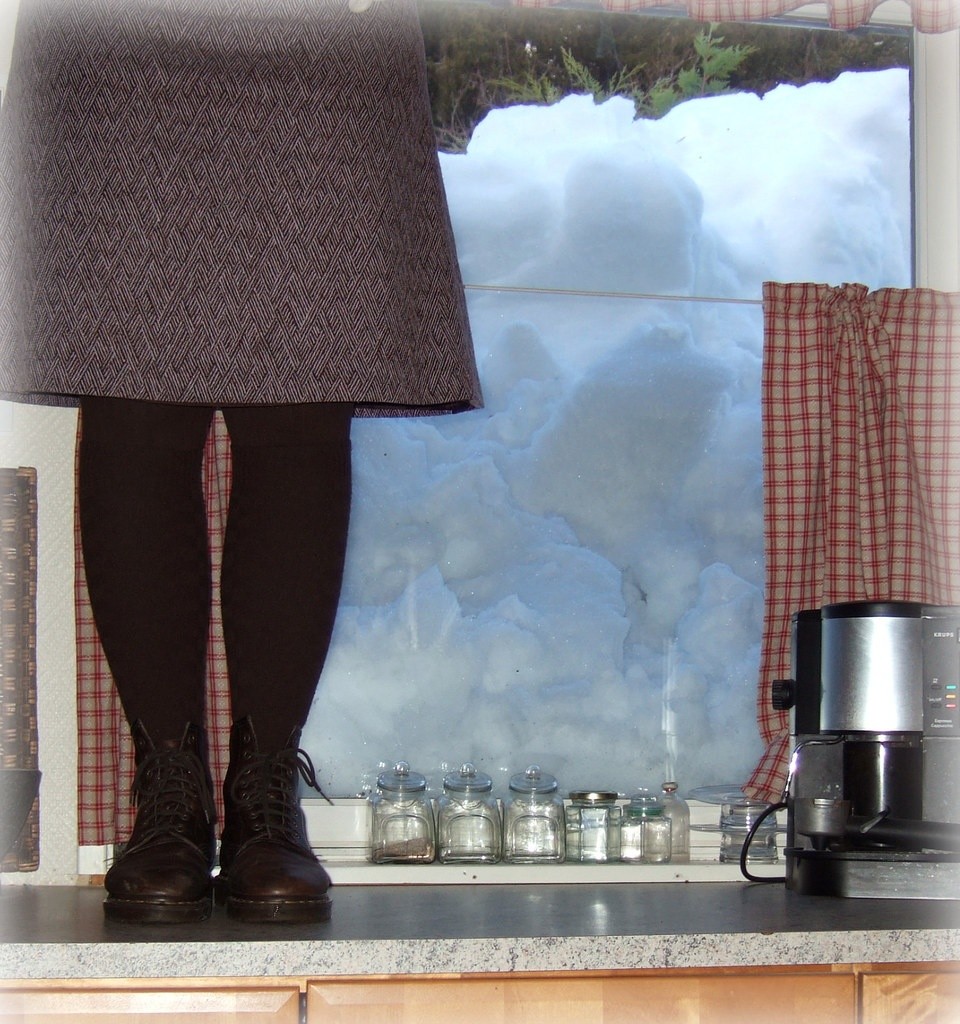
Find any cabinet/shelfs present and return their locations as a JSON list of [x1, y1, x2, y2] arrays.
[[0, 960, 959, 1024]]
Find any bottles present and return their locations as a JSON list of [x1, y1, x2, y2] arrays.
[[661, 782, 691, 864], [504, 766, 565, 863], [432, 764, 502, 864], [368, 761, 436, 864], [621, 794, 672, 863]]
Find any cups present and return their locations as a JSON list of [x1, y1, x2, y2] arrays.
[[719, 802, 778, 865], [564, 791, 622, 863]]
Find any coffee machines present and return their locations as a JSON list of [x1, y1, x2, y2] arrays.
[[771, 600, 960, 899]]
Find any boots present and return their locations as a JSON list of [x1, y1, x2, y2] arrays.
[[220, 718, 333, 922], [102, 719, 215, 922]]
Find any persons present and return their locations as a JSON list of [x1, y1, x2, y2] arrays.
[[2, 0, 485, 926]]
